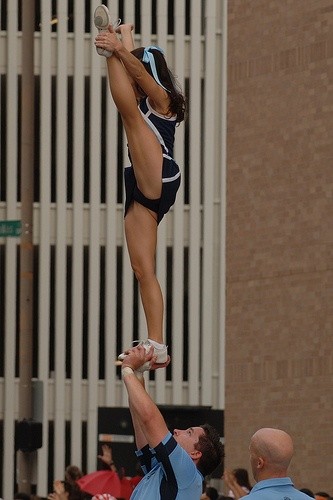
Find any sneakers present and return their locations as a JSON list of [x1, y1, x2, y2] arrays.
[[118, 339, 168, 365], [93, 5, 113, 57]]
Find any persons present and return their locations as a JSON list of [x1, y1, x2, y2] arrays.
[[94, 4, 187, 364], [121, 345, 225, 500], [48, 428, 333, 500]]
